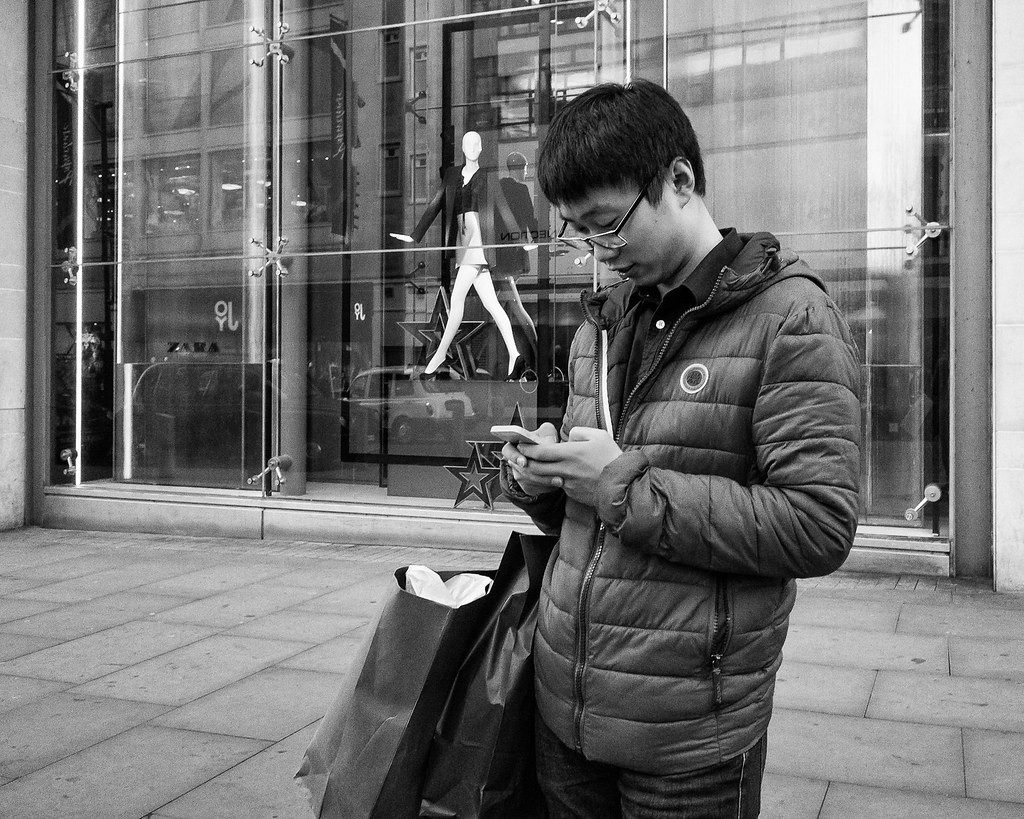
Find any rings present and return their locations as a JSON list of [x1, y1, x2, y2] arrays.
[[551, 476, 564, 487]]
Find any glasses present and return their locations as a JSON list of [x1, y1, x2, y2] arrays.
[[557, 163, 670, 252]]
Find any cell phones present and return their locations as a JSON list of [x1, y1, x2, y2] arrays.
[[490, 425, 541, 448]]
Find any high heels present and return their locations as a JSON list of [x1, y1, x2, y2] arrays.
[[419, 360, 450, 380], [504, 354, 527, 382]]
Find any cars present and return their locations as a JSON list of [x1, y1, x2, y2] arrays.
[[134, 362, 346, 474], [339, 364, 476, 447]]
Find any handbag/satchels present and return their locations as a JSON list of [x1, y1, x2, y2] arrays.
[[292, 563, 497, 819], [414, 531, 561, 819]]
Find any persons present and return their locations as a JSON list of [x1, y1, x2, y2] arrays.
[[482, 152, 567, 379], [499, 79, 862, 819], [390, 131, 539, 381]]
